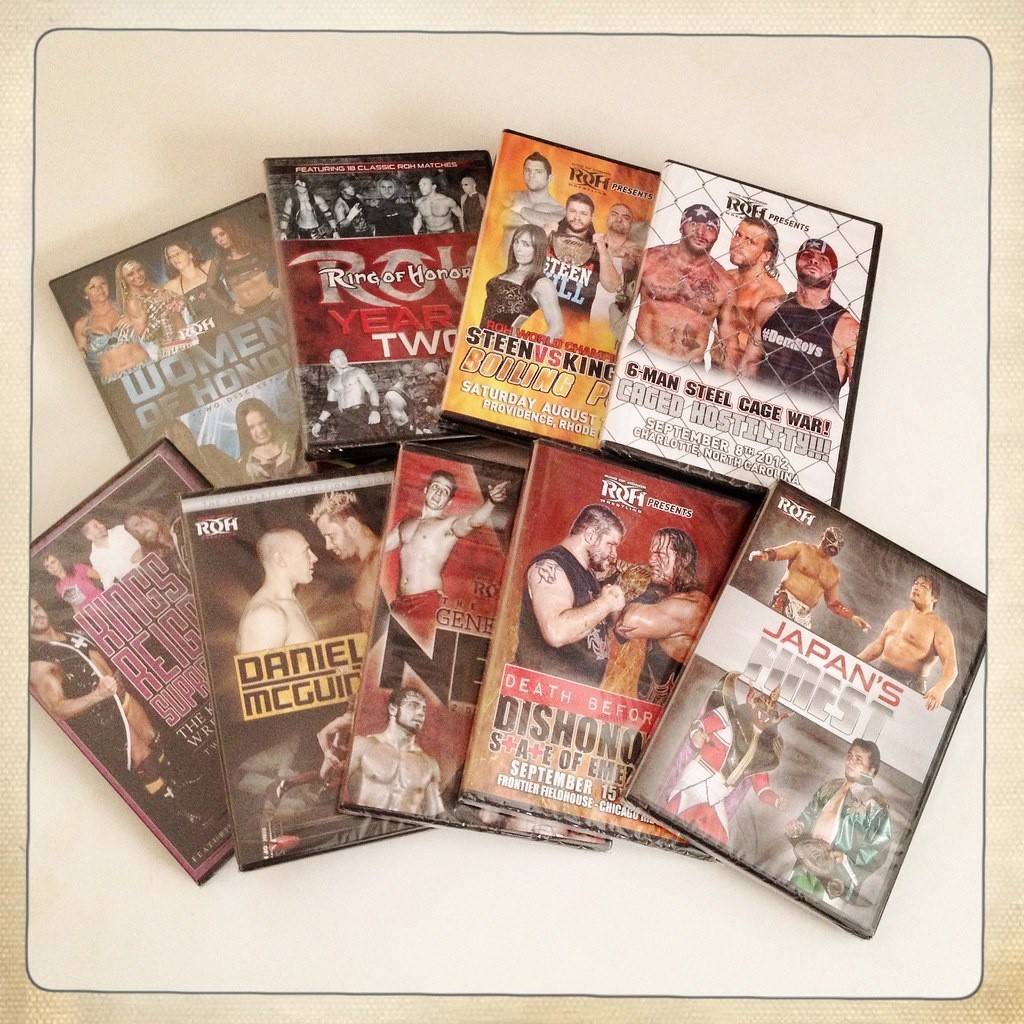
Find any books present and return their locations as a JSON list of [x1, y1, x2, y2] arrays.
[[261, 149, 493, 462], [457, 436, 758, 863], [336, 442, 614, 854], [49, 191, 318, 488], [29, 439, 235, 886], [440, 127, 661, 455], [623, 478, 987, 942], [598, 156, 883, 512], [176, 464, 434, 874]]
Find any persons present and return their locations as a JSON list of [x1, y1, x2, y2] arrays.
[[312, 350, 380, 442], [29, 503, 215, 829], [71, 151, 861, 481], [784, 737, 894, 910], [380, 469, 513, 642], [514, 503, 710, 705], [420, 361, 449, 420], [234, 489, 380, 655], [748, 528, 873, 640], [856, 573, 959, 711], [382, 361, 433, 439], [347, 686, 448, 819]]
